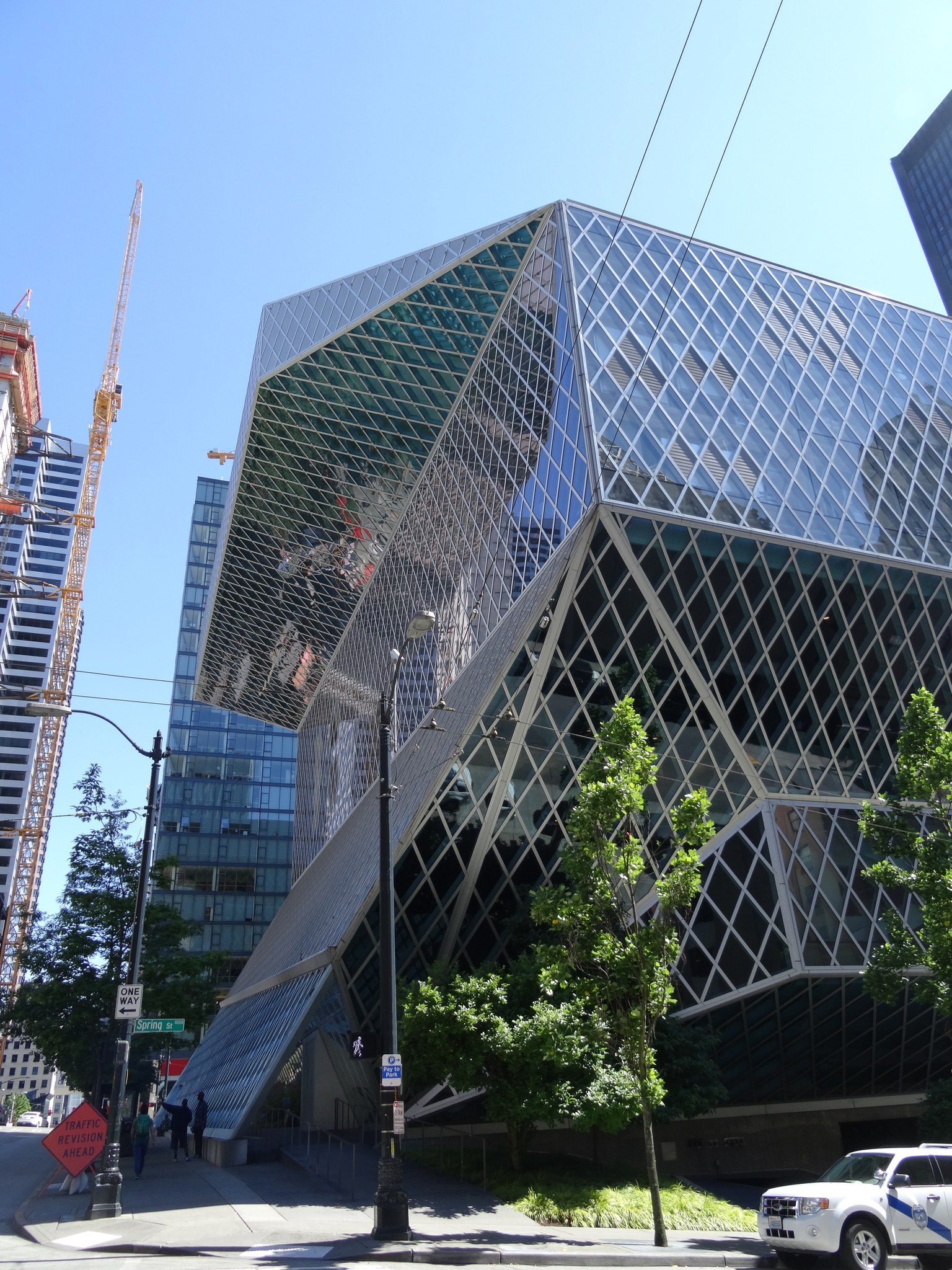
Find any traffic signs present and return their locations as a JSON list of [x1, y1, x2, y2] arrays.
[[114, 984, 143, 1018], [383, 1054, 402, 1087]]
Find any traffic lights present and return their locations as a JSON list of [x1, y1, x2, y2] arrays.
[[48, 1110, 51, 1116], [349, 1033, 379, 1061]]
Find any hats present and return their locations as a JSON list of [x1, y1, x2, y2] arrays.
[[196, 1092, 205, 1097]]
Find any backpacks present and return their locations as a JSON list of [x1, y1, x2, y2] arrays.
[[136, 1114, 150, 1136]]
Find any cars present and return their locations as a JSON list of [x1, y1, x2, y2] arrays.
[[16, 1111, 49, 1128], [756, 1143, 952, 1270]]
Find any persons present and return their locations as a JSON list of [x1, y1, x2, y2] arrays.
[[159, 1098, 192, 1162], [130, 1104, 155, 1179], [191, 1092, 208, 1159]]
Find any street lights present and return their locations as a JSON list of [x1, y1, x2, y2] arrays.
[[0, 1076, 30, 1089], [25, 701, 172, 1220], [374, 609, 436, 1244]]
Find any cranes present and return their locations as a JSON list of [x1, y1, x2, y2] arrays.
[[1, 179, 148, 1058], [206, 447, 236, 464]]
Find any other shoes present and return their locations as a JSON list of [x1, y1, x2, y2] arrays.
[[186, 1158, 192, 1161], [139, 1174, 142, 1177], [192, 1154, 201, 1159], [133, 1176, 138, 1180], [173, 1159, 177, 1163]]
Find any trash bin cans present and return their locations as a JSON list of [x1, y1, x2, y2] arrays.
[[119, 1116, 134, 1157]]
